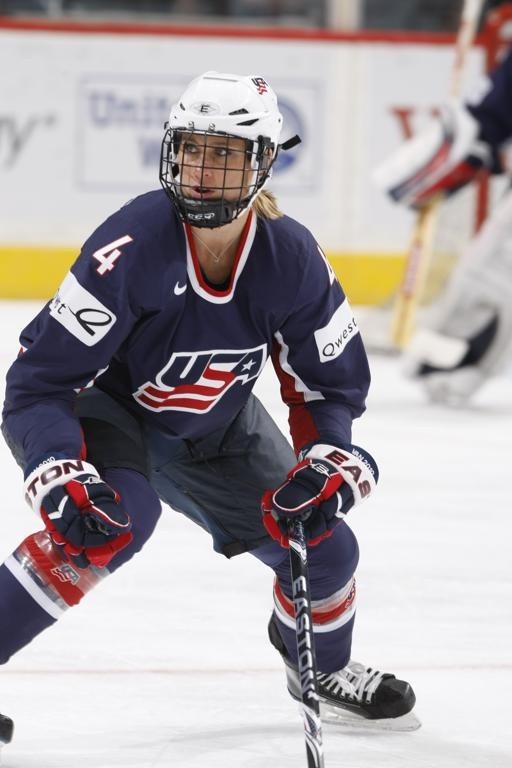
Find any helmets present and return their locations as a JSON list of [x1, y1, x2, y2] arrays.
[[159, 73, 282, 228]]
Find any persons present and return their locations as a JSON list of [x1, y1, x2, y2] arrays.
[[387, 1, 511, 410], [1, 70, 423, 728]]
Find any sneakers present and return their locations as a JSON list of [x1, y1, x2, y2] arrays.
[[288, 661, 415, 720]]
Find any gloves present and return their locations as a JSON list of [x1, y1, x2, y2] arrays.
[[260, 439, 379, 548], [24, 452, 133, 569]]
